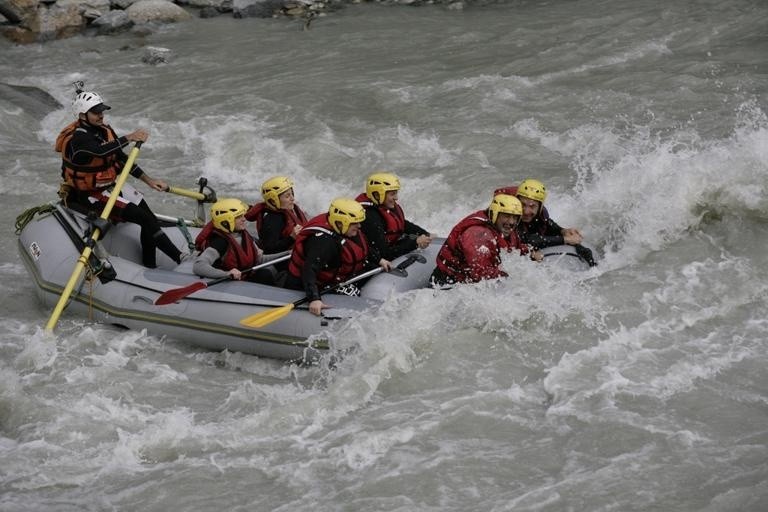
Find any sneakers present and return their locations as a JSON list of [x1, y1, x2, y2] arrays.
[[178, 251, 192, 264]]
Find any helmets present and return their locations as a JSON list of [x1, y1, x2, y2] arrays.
[[71, 92, 112, 119], [487, 193, 523, 228], [366, 173, 401, 204], [262, 176, 294, 212], [326, 199, 367, 235], [514, 179, 547, 217], [210, 198, 249, 233]]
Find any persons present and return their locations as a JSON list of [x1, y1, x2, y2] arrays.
[[55, 91, 190, 270], [354, 173, 437, 261], [193, 197, 290, 288], [243, 175, 310, 255], [493, 179, 584, 263], [286, 198, 393, 317], [428, 194, 544, 291]]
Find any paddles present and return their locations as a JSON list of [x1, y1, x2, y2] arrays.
[[154, 254, 293, 305], [240, 266, 390, 330]]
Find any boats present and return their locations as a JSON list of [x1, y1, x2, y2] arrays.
[[14, 198, 601, 363]]
